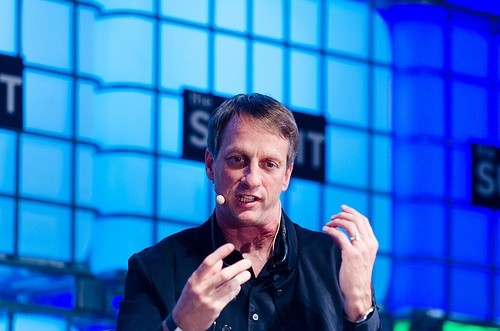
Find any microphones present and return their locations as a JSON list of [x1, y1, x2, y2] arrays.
[[214, 185, 226, 206]]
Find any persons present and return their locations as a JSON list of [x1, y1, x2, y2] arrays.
[[114, 93, 384, 331]]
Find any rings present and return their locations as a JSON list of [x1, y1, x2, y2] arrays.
[[349, 233, 361, 242]]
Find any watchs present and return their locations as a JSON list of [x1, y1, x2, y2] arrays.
[[342, 305, 375, 323]]
